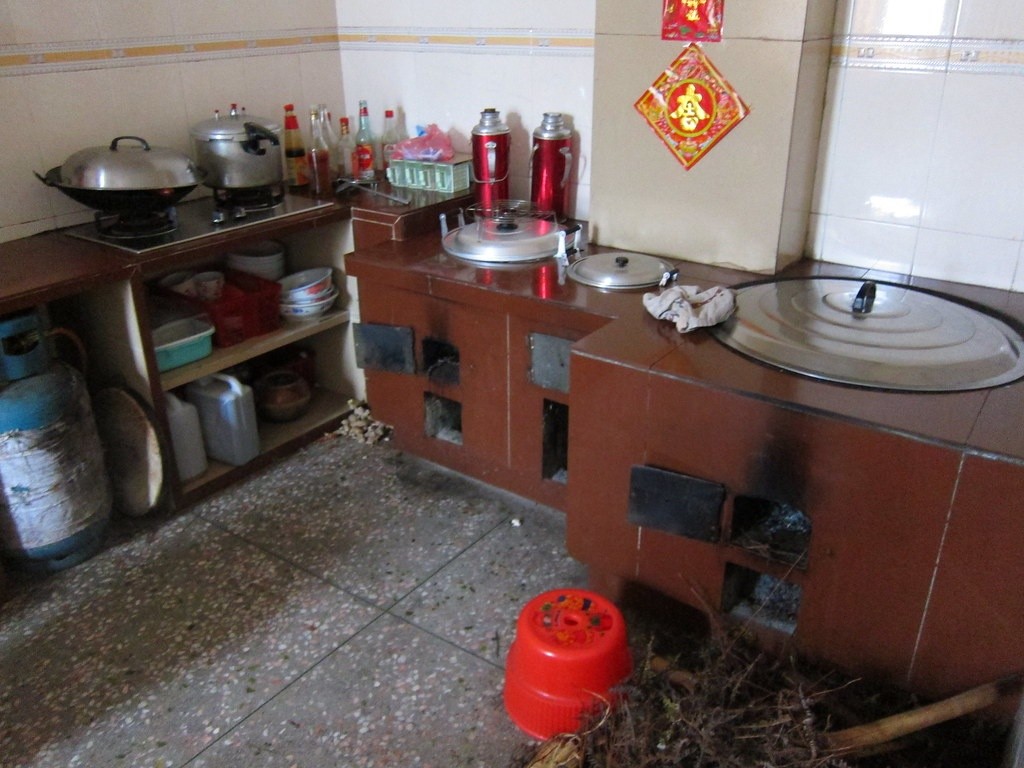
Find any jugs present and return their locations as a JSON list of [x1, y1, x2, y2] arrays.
[[466, 108, 517, 218], [527, 111, 574, 223]]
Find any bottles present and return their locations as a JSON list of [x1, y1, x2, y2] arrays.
[[380, 110, 400, 178], [337, 115, 356, 181], [353, 99, 376, 180], [281, 103, 310, 194], [318, 100, 340, 183], [307, 105, 328, 196], [249, 362, 312, 424]]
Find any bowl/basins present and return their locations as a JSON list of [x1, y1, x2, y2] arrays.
[[275, 269, 333, 301], [226, 238, 285, 280], [279, 288, 339, 321], [279, 284, 334, 305], [162, 270, 226, 303]]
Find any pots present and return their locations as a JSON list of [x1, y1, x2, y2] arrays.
[[32, 134, 211, 216], [186, 103, 286, 192]]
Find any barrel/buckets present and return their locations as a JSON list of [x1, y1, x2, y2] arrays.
[[163, 390, 209, 483], [183, 373, 261, 467]]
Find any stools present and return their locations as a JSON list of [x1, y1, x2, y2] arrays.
[[502, 588, 632, 743]]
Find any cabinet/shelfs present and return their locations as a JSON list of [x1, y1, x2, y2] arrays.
[[1, 171, 367, 520]]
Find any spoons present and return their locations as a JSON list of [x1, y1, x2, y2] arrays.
[[333, 175, 411, 206]]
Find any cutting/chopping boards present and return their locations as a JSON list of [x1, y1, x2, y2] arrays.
[[94, 384, 171, 520]]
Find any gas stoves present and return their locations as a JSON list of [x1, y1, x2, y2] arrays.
[[63, 189, 335, 254]]
